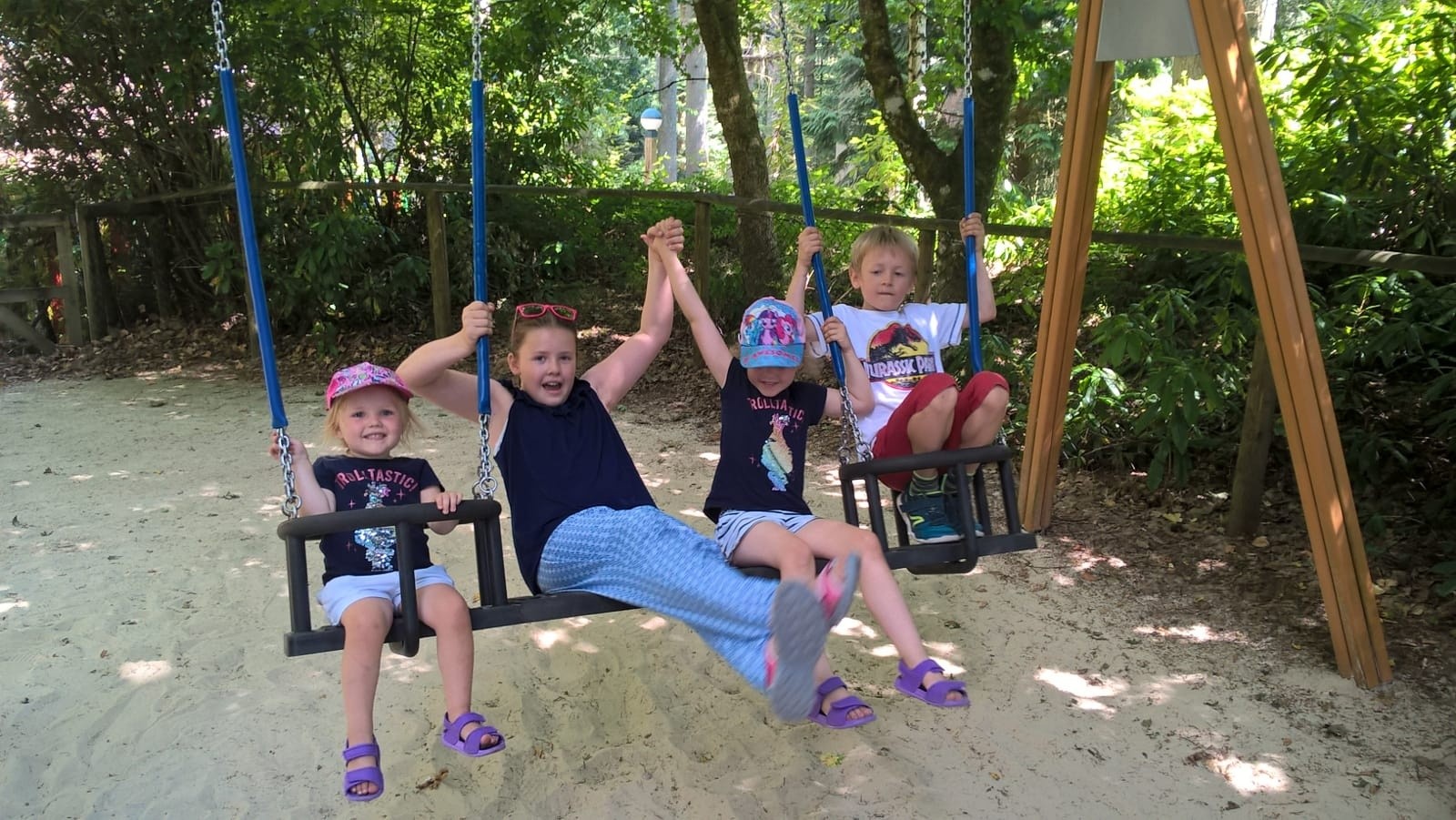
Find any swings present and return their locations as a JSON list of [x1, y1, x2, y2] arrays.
[[207, 0, 1047, 662]]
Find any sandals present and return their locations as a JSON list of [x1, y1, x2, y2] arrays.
[[442, 712, 505, 756], [894, 658, 971, 706], [806, 676, 877, 728], [342, 735, 384, 801]]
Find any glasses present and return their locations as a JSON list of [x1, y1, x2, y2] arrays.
[[511, 303, 577, 343]]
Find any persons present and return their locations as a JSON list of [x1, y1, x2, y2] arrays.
[[395, 218, 862, 727], [786, 211, 1010, 545], [641, 218, 971, 727], [269, 362, 507, 803]]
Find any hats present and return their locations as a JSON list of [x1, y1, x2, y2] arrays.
[[327, 362, 414, 410], [739, 296, 807, 367]]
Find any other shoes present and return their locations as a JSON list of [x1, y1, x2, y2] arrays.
[[763, 580, 828, 722], [815, 554, 860, 628]]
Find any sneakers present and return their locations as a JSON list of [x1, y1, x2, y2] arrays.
[[939, 473, 985, 537], [897, 481, 960, 543]]
